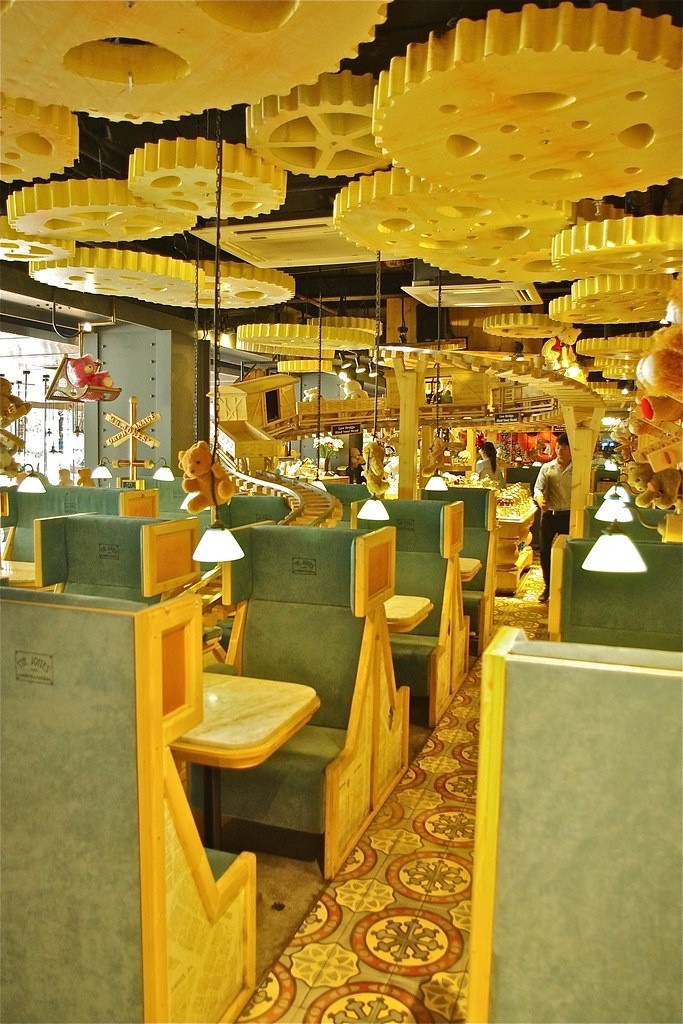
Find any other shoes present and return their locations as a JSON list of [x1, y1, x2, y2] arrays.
[[538, 589, 549, 601]]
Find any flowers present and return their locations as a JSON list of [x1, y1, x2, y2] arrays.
[[312, 436, 346, 457]]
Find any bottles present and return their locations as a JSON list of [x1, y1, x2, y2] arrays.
[[442, 471, 533, 519], [277, 455, 327, 484]]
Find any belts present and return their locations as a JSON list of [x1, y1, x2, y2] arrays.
[[546, 510, 570, 517]]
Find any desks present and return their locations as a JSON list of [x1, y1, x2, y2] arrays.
[[382, 595, 434, 633], [168, 671, 322, 856], [456, 556, 484, 585], [0, 559, 54, 590]]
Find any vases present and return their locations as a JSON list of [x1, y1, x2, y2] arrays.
[[324, 457, 330, 472]]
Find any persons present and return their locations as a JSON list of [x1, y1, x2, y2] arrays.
[[476, 442, 509, 481], [544, 441, 553, 455], [534, 434, 572, 601]]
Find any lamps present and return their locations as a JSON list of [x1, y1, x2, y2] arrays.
[[424, 260, 450, 492], [337, 352, 351, 370], [356, 243, 389, 524], [594, 482, 642, 523], [581, 507, 682, 572], [604, 473, 629, 501], [9, 463, 46, 495], [151, 457, 175, 484], [353, 357, 366, 374], [367, 358, 380, 377], [90, 455, 114, 479], [192, 183, 244, 561], [307, 259, 328, 494]]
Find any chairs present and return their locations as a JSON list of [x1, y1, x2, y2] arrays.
[[0, 476, 682, 1020]]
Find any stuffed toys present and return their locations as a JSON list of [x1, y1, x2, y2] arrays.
[[303, 387, 326, 402], [0, 378, 33, 478], [340, 378, 370, 400], [178, 441, 234, 514], [350, 449, 366, 471], [58, 469, 76, 486], [70, 354, 112, 399], [611, 269, 683, 509], [541, 323, 581, 371], [77, 468, 95, 486], [364, 442, 390, 495]]
